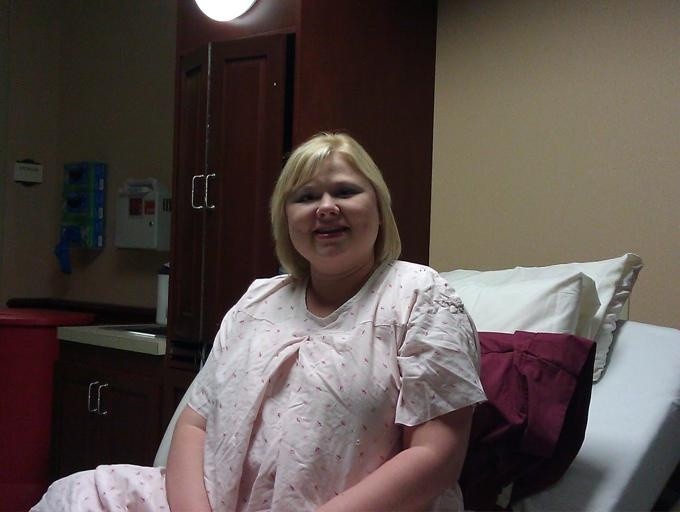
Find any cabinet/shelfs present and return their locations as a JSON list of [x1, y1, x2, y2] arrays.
[[49, 338, 162, 483]]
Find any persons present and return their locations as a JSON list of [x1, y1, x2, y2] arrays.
[[28, 130, 490, 511]]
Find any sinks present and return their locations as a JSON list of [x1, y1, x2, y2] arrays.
[[56, 323, 168, 356]]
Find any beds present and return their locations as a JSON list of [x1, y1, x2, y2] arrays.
[[28, 323, 679, 512]]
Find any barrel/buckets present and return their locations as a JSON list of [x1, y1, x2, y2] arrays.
[[0, 306, 95, 512]]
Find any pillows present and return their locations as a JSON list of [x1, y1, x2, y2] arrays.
[[454, 272, 601, 339], [439, 252, 643, 384]]
